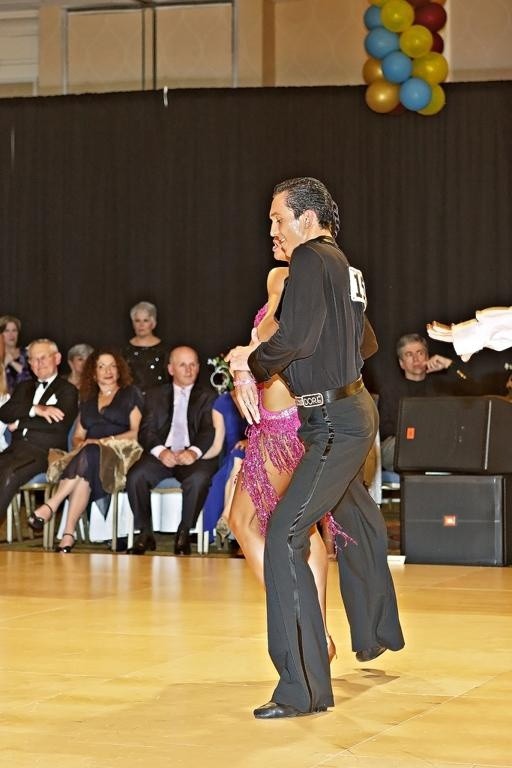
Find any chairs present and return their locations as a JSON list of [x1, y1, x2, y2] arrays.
[[381, 472, 400, 503], [0, 414, 210, 557]]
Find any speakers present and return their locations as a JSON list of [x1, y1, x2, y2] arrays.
[[400, 473, 512, 566], [394, 395, 512, 477]]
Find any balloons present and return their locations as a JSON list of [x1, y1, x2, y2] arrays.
[[363, 1, 448, 116]]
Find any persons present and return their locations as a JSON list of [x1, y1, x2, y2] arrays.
[[427, 306, 512, 361], [322, 445, 377, 559], [230, 201, 340, 676], [224, 177, 406, 718], [1, 300, 250, 558], [377, 333, 468, 471]]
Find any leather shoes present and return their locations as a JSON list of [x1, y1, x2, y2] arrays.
[[57, 531, 77, 553], [134, 531, 156, 551], [355, 646, 386, 662], [253, 699, 328, 719], [28, 503, 53, 533], [176, 526, 190, 550]]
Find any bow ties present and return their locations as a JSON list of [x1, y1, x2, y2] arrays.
[[34, 380, 49, 388]]
